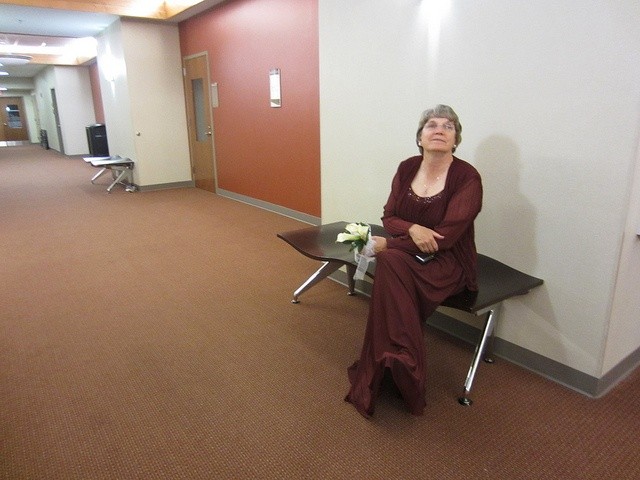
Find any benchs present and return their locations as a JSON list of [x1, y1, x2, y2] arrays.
[[277, 221, 544, 405], [83, 155, 136, 193]]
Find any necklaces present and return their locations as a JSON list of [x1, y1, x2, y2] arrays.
[[413, 158, 450, 195]]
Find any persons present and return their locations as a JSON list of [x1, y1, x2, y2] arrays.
[[344, 105, 482, 420]]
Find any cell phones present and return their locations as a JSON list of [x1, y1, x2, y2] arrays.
[[416, 255, 435, 265]]
